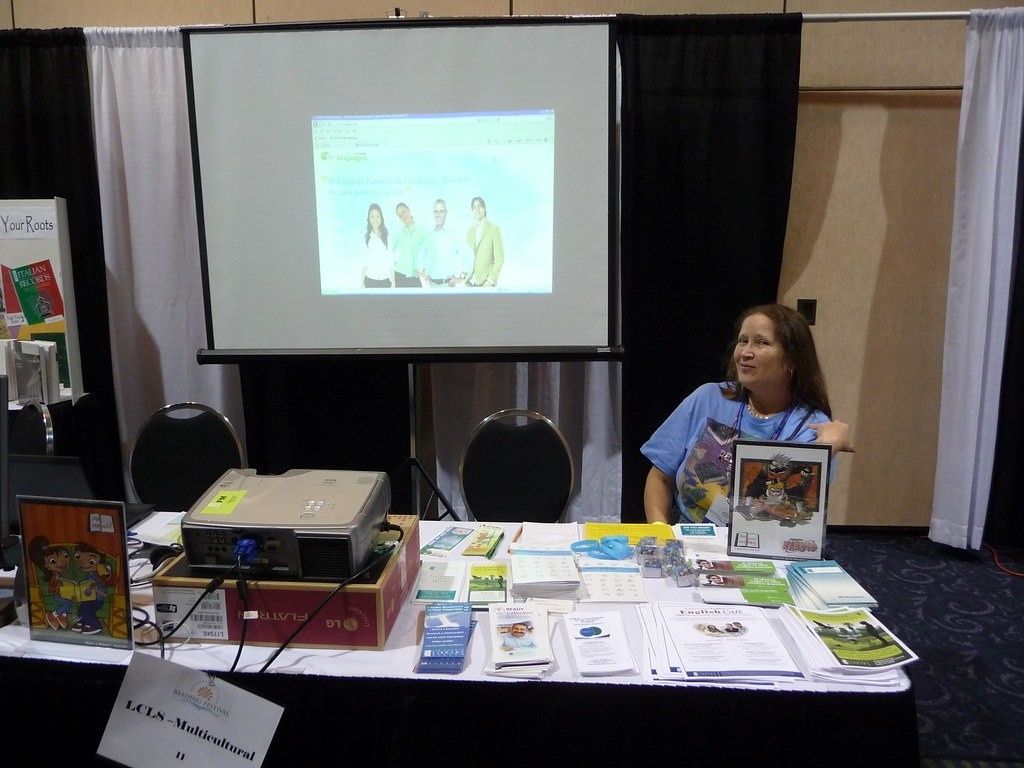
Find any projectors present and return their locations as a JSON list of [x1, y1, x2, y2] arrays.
[[181, 470, 392, 582]]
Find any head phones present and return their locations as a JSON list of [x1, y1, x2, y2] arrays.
[[128, 545, 179, 583]]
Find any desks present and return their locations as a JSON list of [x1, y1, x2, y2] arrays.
[[1, 518, 916, 767]]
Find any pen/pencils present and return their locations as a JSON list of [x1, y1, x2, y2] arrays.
[[508, 527, 523, 554], [486, 530, 505, 560]]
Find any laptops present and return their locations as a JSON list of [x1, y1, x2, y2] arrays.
[[7, 453, 155, 535]]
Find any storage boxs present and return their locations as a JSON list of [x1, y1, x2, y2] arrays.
[[152, 514, 415, 651]]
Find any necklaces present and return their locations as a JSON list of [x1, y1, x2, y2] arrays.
[[748, 404, 770, 419]]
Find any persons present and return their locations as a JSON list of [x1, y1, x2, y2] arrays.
[[696, 559, 713, 570], [500, 623, 535, 651], [418, 199, 474, 288], [860, 620, 889, 646], [704, 575, 724, 586], [390, 203, 426, 288], [640, 305, 856, 526], [464, 196, 504, 286], [360, 203, 393, 288], [697, 622, 744, 634]]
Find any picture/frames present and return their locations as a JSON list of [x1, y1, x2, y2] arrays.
[[727, 437, 833, 560]]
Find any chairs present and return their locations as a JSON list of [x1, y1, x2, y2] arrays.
[[459, 408, 575, 522], [128, 400, 245, 512], [9, 397, 55, 455]]
